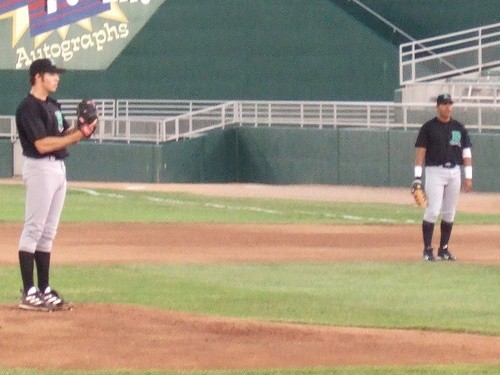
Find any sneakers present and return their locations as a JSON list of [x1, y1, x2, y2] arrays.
[[40, 285, 74, 313], [439, 245, 457, 262], [422, 245, 437, 263], [18, 288, 50, 312]]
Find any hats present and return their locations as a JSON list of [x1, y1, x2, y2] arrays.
[[28, 58, 65, 76], [436, 93, 454, 105]]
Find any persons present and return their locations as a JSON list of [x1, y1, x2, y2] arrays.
[[411, 94, 473, 261], [16, 58, 98, 310]]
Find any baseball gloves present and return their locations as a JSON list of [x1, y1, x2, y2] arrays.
[[77, 97, 97, 129], [413, 188, 428, 208]]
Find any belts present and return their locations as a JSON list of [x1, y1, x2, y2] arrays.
[[439, 163, 456, 168]]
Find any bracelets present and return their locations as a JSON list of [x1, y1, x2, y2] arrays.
[[414, 166, 422, 176], [465, 166, 472, 179]]
